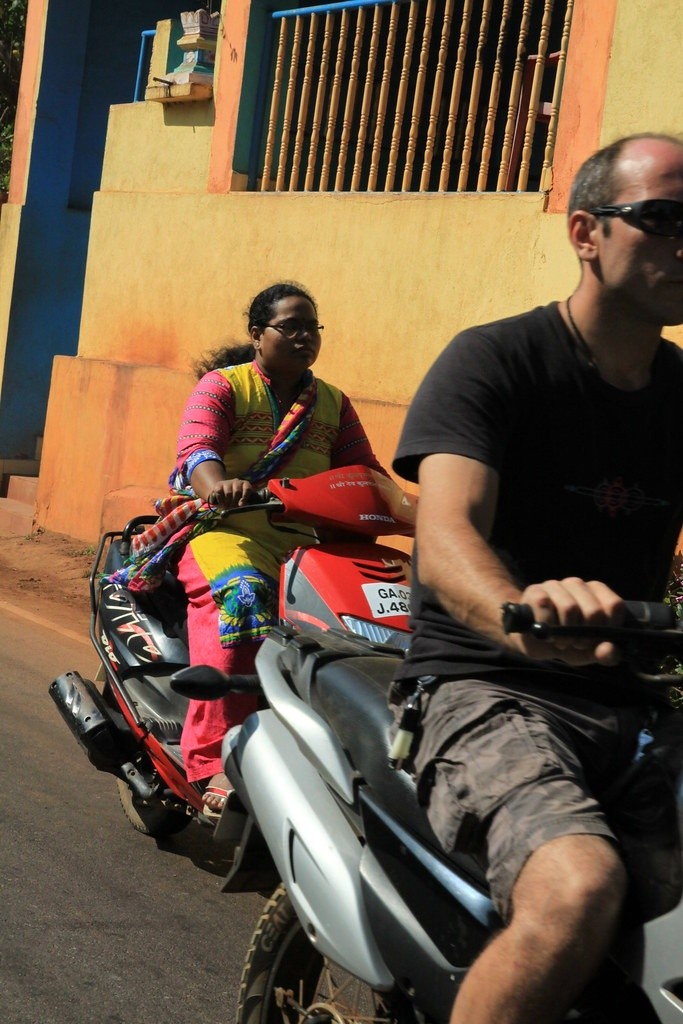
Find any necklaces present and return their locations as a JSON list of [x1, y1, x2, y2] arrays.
[[567, 296, 601, 374]]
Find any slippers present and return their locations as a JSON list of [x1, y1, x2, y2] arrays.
[[203, 786, 235, 817]]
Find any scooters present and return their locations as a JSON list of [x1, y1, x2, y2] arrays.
[[49, 466, 419, 848], [169, 602, 683, 1024]]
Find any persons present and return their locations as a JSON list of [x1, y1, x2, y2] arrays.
[[161, 281, 391, 820], [392, 132, 683, 1024]]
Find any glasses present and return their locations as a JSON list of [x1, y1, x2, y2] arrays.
[[262, 322, 323, 337], [586, 199, 683, 237]]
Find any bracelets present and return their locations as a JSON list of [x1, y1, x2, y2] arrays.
[[207, 490, 221, 516]]
